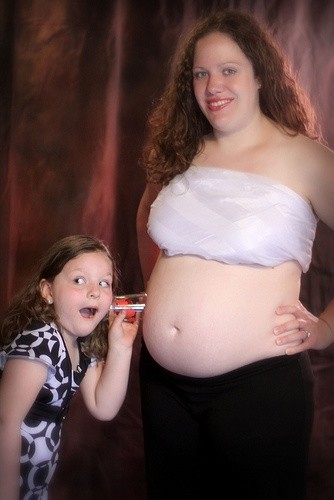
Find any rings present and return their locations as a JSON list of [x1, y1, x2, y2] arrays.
[[305, 331, 310, 338]]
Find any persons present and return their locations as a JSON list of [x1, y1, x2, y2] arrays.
[[136, 10, 334, 499], [0, 236, 141, 500]]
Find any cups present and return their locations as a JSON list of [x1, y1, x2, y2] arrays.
[[109, 293, 149, 321]]
